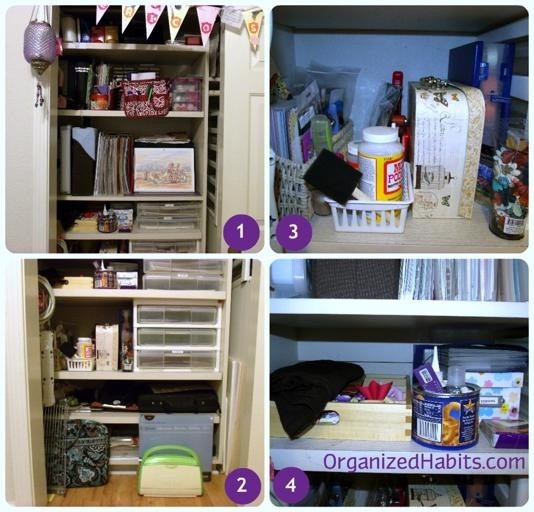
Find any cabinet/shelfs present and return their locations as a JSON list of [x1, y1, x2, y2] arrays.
[[57, 7, 207, 253], [39, 259, 233, 476], [269, 259, 529, 507]]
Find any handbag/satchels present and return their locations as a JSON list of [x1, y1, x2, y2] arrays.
[[45, 419, 110, 488]]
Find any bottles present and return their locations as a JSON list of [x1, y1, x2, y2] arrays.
[[92, 270, 114, 289], [96, 213, 117, 233], [386, 70, 408, 130], [101, 204, 108, 218], [76, 336, 91, 360], [346, 126, 405, 225], [445, 365, 466, 393]]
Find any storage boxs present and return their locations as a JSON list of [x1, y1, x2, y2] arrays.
[[268, 373, 415, 442]]
